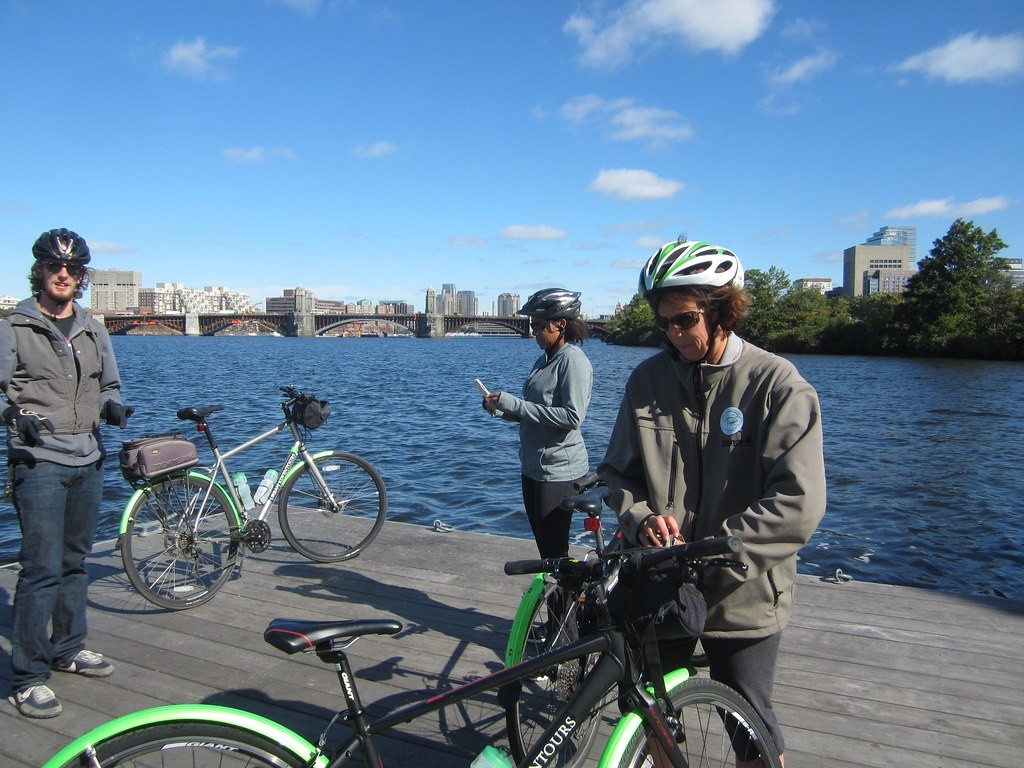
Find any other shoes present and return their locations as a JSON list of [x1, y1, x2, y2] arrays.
[[528, 664, 563, 681], [527, 622, 548, 643]]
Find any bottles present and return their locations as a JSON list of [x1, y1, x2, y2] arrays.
[[468, 744, 512, 768], [254, 469, 279, 504], [232, 471, 255, 510]]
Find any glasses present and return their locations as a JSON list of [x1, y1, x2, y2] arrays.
[[531, 320, 552, 330], [42, 261, 81, 275], [652, 308, 709, 331]]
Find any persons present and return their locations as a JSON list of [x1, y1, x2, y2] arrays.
[[596, 234, 828, 768], [480, 288, 594, 643], [0, 227, 135, 719]]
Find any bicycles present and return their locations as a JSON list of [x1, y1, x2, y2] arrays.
[[40, 530, 787, 768], [500, 473, 711, 768], [115, 385, 388, 612]]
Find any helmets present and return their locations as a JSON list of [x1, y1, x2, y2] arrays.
[[32, 228, 91, 265], [638, 240, 744, 299], [517, 288, 581, 319]]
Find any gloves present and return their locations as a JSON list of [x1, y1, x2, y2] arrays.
[[4, 406, 55, 446], [105, 398, 135, 428]]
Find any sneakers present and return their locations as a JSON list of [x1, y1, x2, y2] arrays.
[[56, 649, 115, 678], [7, 684, 62, 718]]
